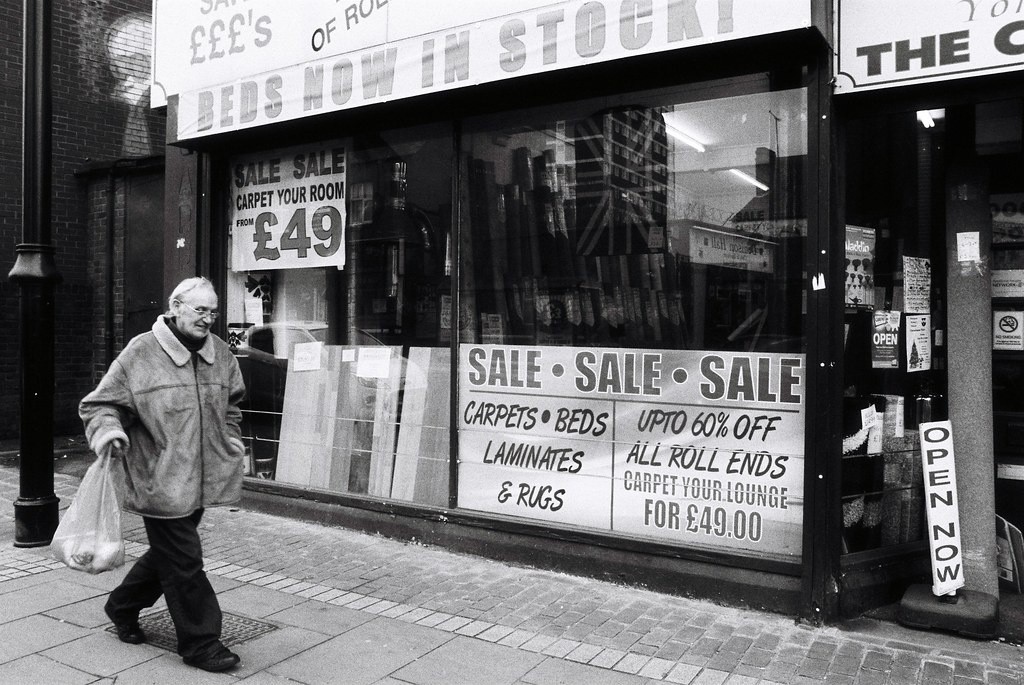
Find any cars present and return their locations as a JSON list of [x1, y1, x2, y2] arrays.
[[232, 322, 431, 423]]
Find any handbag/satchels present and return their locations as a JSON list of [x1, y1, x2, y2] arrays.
[[50, 444, 124, 574]]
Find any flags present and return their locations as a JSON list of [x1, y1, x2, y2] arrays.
[[573, 111, 671, 257]]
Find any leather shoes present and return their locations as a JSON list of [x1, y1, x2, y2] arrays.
[[103, 598, 147, 644], [183, 646, 240, 671]]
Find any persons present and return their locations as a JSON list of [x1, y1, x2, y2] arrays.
[[78, 275, 247, 673]]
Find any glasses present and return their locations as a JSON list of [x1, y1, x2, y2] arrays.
[[179, 300, 221, 319]]
